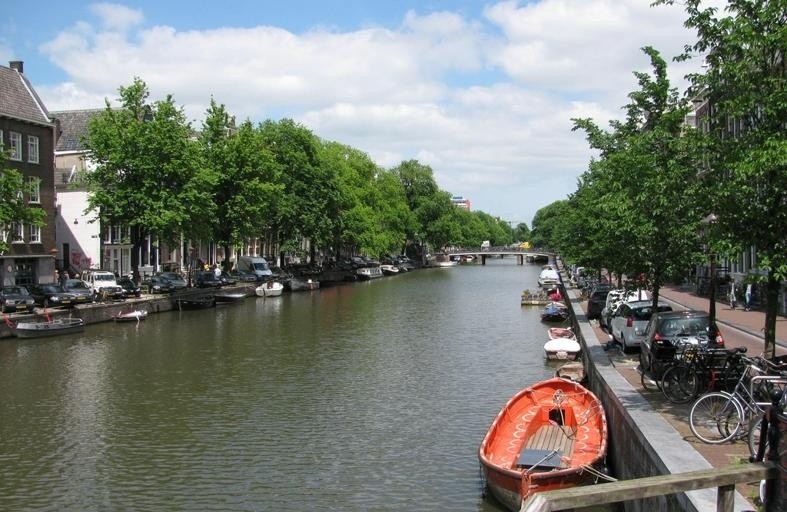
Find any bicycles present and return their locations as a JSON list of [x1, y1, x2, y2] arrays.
[[641, 336, 787, 459]]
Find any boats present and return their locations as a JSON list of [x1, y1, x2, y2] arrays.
[[519, 266, 579, 363], [473, 374, 612, 510], [112, 309, 148, 324], [175, 297, 219, 311], [197, 292, 249, 303], [454, 255, 479, 261], [251, 280, 285, 297], [526, 255, 540, 264], [553, 359, 587, 385], [430, 260, 458, 267], [13, 314, 90, 341]]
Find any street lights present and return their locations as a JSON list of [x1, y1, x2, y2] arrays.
[[708, 249, 720, 326]]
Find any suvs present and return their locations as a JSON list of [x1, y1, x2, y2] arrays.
[[26, 281, 78, 309], [78, 267, 123, 302], [637, 307, 727, 377]]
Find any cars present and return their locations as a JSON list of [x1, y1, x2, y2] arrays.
[[769, 385, 787, 511], [114, 275, 141, 299], [568, 265, 674, 348], [0, 284, 36, 313], [146, 254, 410, 291], [61, 279, 91, 302]]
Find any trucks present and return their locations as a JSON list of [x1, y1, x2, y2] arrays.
[[520, 241, 532, 249]]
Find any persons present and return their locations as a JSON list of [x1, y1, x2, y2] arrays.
[[726, 277, 739, 309], [742, 283, 756, 312]]
